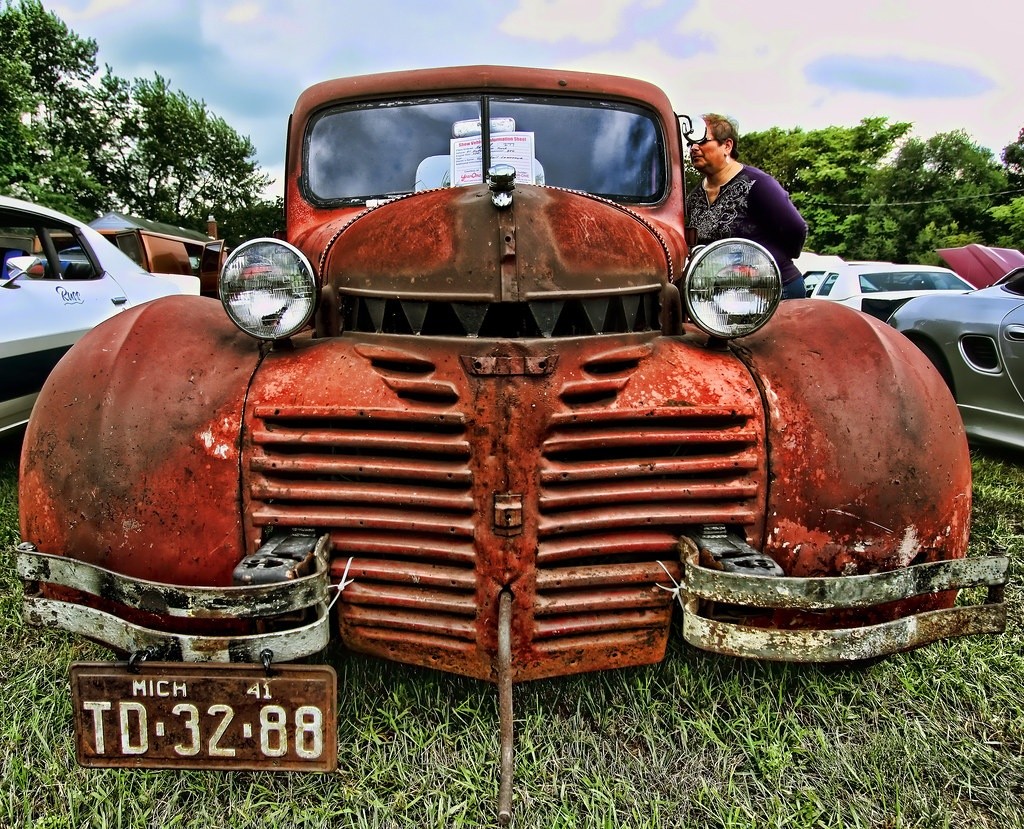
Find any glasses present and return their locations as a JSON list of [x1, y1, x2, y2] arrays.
[[687, 138, 717, 147]]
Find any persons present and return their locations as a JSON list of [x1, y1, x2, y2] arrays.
[[687, 113, 807, 301]]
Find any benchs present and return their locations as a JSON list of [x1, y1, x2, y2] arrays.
[[39, 259, 94, 280]]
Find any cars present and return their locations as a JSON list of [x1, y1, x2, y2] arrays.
[[13, 63, 1013, 783], [0, 194, 202, 437], [709, 286, 773, 331], [224, 286, 310, 336], [781, 245, 1024, 450]]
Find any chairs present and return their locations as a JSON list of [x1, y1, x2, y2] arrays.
[[0, 246, 30, 280]]
